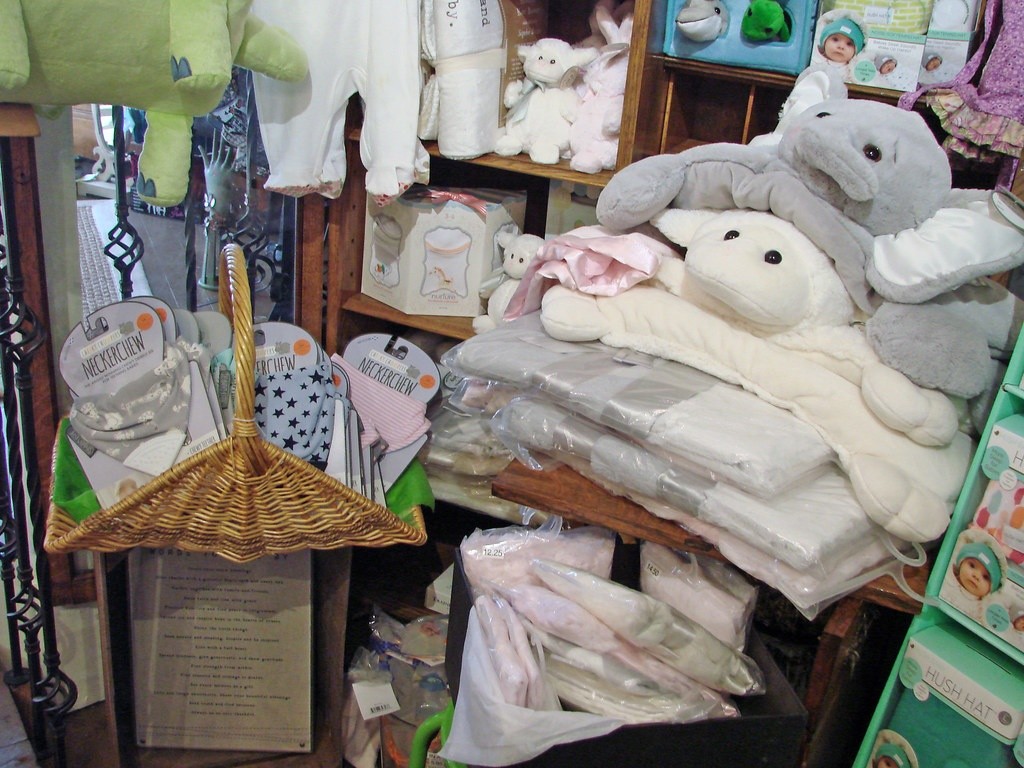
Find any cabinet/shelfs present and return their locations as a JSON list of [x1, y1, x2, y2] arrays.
[[323, 0, 1024, 768]]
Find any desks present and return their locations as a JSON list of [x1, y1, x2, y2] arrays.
[[490, 443, 932, 768]]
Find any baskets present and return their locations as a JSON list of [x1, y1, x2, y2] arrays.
[[43, 242, 428, 562]]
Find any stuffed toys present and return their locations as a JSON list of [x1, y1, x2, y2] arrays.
[[472, 227, 547, 335], [677, 0, 728, 44], [496, 0, 634, 176], [541, 61, 1024, 542], [0, 0, 310, 209], [740, 0, 792, 43]]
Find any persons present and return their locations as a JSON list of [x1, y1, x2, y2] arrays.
[[870, 743, 912, 768], [940, 541, 1002, 617], [922, 49, 943, 72], [873, 53, 898, 75], [813, 18, 865, 83], [1008, 603, 1024, 632]]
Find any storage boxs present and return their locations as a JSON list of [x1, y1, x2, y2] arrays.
[[360, 185, 529, 318], [544, 178, 603, 235], [899, 312, 1024, 767], [810, 7, 977, 94]]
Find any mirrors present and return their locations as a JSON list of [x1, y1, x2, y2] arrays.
[[9, 67, 323, 609]]
